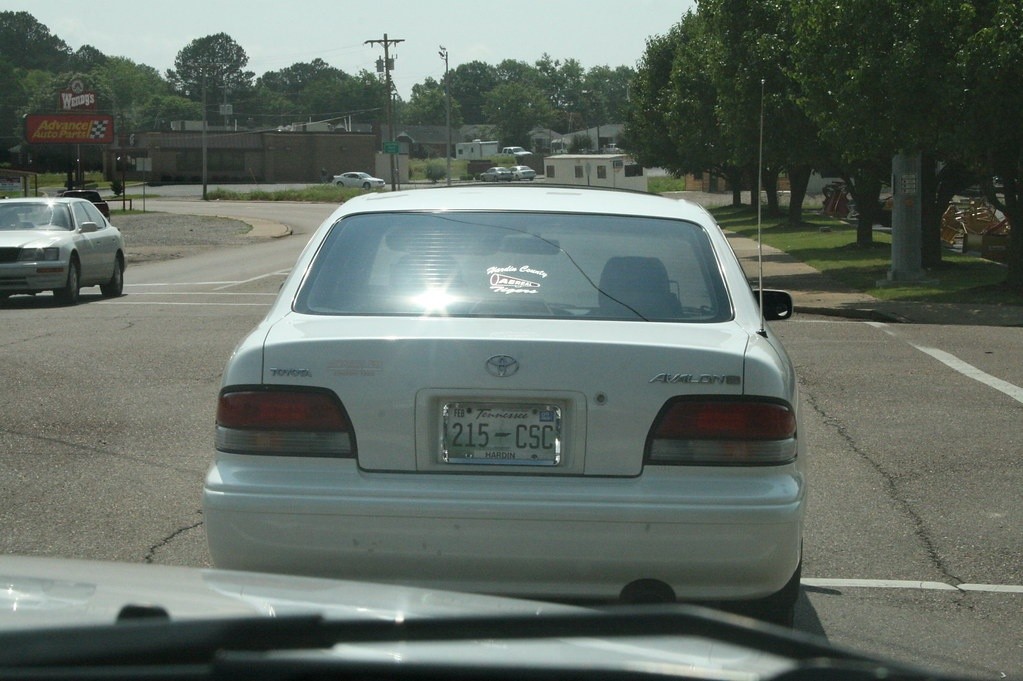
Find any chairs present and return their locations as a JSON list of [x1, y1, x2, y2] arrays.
[[598, 256, 672, 313], [393, 250, 471, 299]]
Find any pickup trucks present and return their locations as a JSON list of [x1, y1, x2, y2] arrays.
[[60, 189, 111, 223], [493, 145, 534, 162]]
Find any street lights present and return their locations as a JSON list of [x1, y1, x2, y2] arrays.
[[115, 112, 126, 210], [224, 67, 240, 131], [437, 43, 453, 186]]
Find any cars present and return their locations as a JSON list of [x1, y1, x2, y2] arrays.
[[331, 171, 386, 190], [202, 185, 803, 633], [601, 143, 624, 154], [879, 191, 1009, 249], [0, 196, 126, 308], [480, 166, 513, 182], [508, 166, 537, 182]]
